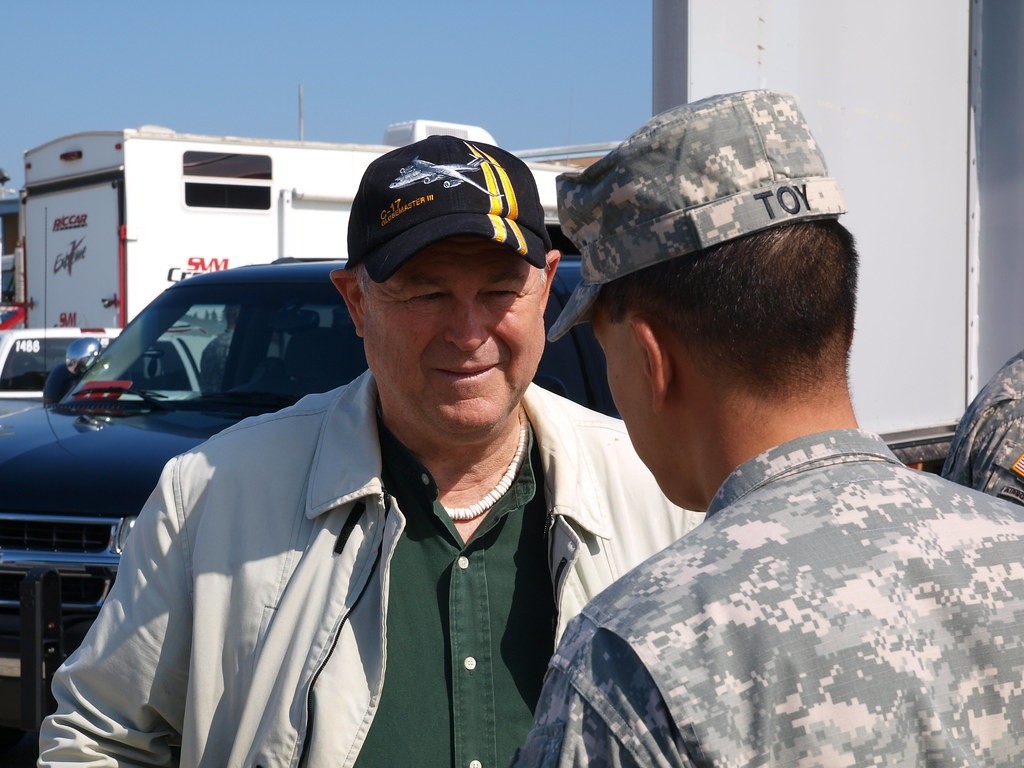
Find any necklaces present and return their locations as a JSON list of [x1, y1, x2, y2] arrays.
[[444, 401, 529, 520]]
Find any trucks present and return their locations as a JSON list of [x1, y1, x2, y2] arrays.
[[21, 118, 629, 328]]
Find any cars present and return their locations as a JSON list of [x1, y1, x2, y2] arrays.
[[0, 258, 624, 742]]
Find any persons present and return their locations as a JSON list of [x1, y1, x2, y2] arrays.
[[941, 350, 1024, 506], [517, 88, 1024, 768], [201, 303, 278, 389], [37, 134, 707, 768]]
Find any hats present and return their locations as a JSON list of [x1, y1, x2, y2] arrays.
[[344, 134, 553, 283], [547, 89, 850, 343]]
